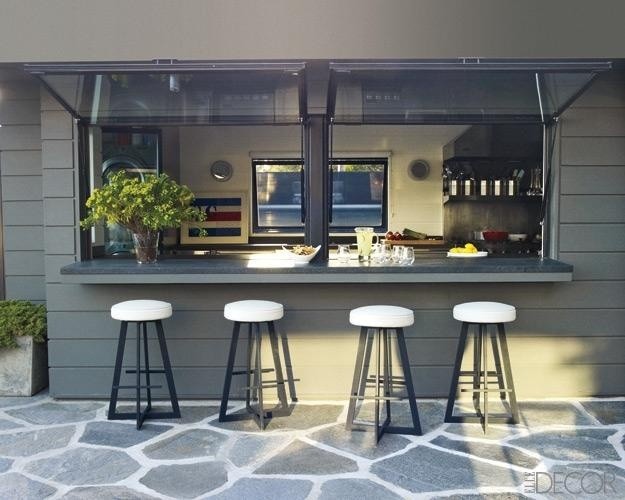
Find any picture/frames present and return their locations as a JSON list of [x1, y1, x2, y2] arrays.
[[181, 191, 250, 244]]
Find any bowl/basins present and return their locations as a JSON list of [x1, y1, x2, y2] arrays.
[[280, 245, 322, 264], [508, 234, 528, 241], [482, 231, 508, 240]]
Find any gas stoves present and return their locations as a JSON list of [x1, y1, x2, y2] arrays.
[[447, 240, 540, 257]]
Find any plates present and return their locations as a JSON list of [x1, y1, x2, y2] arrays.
[[447, 251, 488, 257]]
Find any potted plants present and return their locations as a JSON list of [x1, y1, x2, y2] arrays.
[[80, 169, 208, 264]]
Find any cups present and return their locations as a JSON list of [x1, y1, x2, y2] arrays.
[[380, 244, 391, 261], [392, 246, 405, 263], [336, 245, 351, 264], [354, 227, 379, 262], [400, 247, 414, 265]]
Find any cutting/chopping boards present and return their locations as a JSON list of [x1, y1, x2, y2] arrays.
[[383, 239, 443, 245]]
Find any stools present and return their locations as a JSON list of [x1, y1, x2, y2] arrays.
[[219, 300, 290, 431], [107, 299, 181, 431], [345, 305, 422, 445], [444, 301, 520, 436]]
